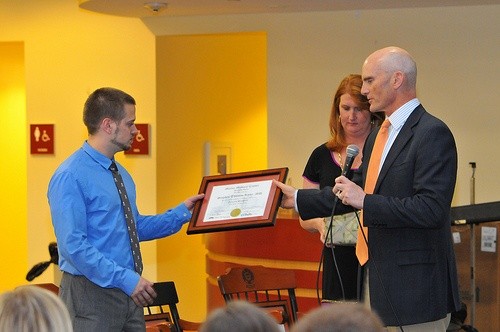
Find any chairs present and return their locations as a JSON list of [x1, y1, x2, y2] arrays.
[[216, 265, 298, 332], [141, 282, 183, 332]]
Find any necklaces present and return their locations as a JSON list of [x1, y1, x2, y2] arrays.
[[336, 146, 362, 172]]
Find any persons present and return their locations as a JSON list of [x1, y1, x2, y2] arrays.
[[298, 71, 387, 304], [271, 46, 463, 332], [0, 284, 75, 332], [198, 298, 281, 332], [286, 299, 388, 332], [46, 86, 207, 331]]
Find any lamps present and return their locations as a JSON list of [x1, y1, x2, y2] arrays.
[[144, 2, 166, 15]]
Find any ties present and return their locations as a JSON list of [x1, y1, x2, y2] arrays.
[[355, 119, 391, 266], [109, 162, 143, 277]]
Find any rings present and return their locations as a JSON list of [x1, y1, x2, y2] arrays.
[[338, 192, 344, 198]]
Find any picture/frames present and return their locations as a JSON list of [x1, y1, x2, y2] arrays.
[[186, 167, 289, 235]]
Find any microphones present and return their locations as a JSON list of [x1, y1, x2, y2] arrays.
[[334, 144, 360, 205]]
